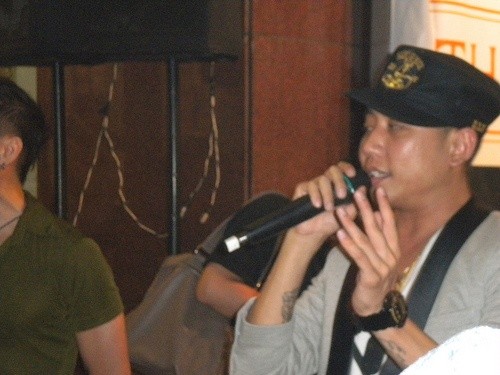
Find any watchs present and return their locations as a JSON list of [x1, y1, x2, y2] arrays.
[[356, 290, 410, 331]]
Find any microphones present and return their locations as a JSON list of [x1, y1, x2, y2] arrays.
[[221, 163, 374, 258]]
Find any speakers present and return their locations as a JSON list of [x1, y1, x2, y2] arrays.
[[1, 0, 241, 62]]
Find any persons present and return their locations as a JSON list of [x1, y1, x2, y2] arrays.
[[196, 193, 328, 336], [1, 72, 133, 374], [227, 42, 499, 375]]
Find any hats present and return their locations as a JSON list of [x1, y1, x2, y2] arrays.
[[343, 44, 498, 127]]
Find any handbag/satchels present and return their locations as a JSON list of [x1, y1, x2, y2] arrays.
[[119, 189, 292, 374]]
